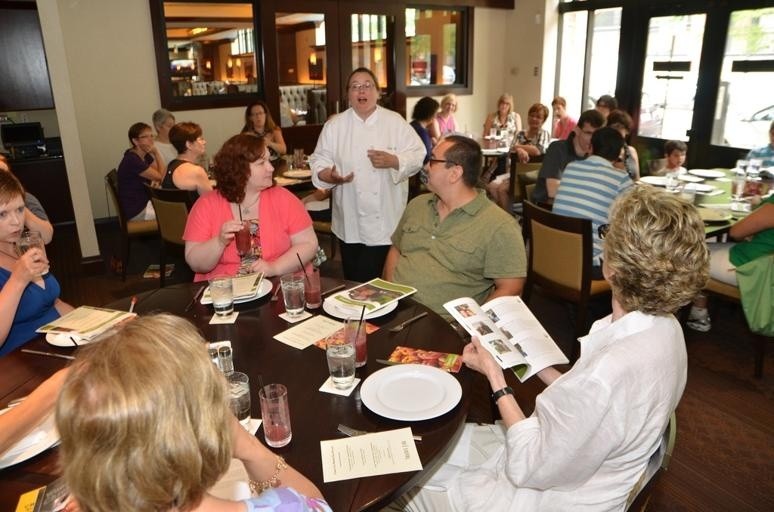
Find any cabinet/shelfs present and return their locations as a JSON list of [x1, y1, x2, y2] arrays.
[[0, 0, 55, 111], [0, 164, 75, 229]]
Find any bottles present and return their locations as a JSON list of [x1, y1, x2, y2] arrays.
[[208, 349, 221, 374], [219, 346, 235, 378]]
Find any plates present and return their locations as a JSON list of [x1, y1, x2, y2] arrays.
[[640, 176, 678, 186], [688, 168, 727, 178], [284, 168, 315, 180], [689, 207, 732, 223], [45, 312, 130, 347], [360, 364, 464, 422], [1, 407, 63, 468], [203, 278, 273, 305], [675, 175, 705, 182], [323, 290, 399, 320]]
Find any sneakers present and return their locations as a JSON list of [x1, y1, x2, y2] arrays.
[[687, 313, 711, 331]]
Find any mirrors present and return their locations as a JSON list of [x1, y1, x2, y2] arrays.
[[148, 0, 262, 110], [405, 6, 475, 98], [261, 0, 341, 156], [340, 1, 405, 124]]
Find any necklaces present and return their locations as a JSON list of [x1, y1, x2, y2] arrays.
[[234, 194, 262, 215]]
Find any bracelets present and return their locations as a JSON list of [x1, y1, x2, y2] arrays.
[[247, 453, 288, 496]]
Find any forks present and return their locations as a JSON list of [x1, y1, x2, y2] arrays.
[[271, 282, 281, 301], [337, 425, 422, 444], [389, 311, 427, 333]]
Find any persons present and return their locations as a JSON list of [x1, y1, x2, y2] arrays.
[[687, 193, 773, 335], [35, 314, 325, 512], [161, 122, 213, 195], [183, 133, 318, 279], [483, 93, 522, 137], [531, 109, 607, 207], [657, 140, 688, 177], [550, 95, 577, 140], [151, 110, 178, 171], [393, 187, 710, 511], [595, 95, 615, 114], [0, 170, 87, 355], [487, 102, 551, 215], [553, 127, 634, 281], [241, 102, 287, 159], [0, 148, 53, 245], [115, 122, 166, 221], [436, 93, 459, 137], [383, 135, 527, 337], [306, 68, 428, 280], [745, 119, 774, 168], [609, 111, 641, 179], [402, 95, 439, 155]]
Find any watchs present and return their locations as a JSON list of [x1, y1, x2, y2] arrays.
[[491, 387, 515, 403]]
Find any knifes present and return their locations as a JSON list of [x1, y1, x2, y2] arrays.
[[22, 349, 76, 360], [185, 285, 203, 312], [322, 284, 346, 296], [376, 358, 452, 374]]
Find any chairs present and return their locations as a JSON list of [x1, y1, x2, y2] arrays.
[[518, 201, 613, 340], [105, 168, 167, 280], [699, 272, 771, 378], [509, 153, 551, 217], [143, 182, 200, 287], [305, 187, 337, 261]]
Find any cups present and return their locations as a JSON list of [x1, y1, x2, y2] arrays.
[[208, 274, 234, 317], [345, 316, 366, 368], [295, 149, 304, 168], [221, 372, 252, 433], [287, 155, 293, 170], [301, 267, 322, 309], [502, 127, 508, 142], [666, 172, 680, 193], [749, 158, 762, 182], [737, 159, 747, 179], [680, 186, 695, 205], [326, 332, 356, 390], [732, 175, 746, 200], [258, 384, 292, 448], [14, 231, 49, 279], [279, 273, 305, 317], [490, 129, 496, 142]]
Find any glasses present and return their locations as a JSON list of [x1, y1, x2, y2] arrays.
[[348, 81, 375, 91], [250, 111, 264, 116], [426, 153, 458, 168], [581, 129, 592, 136], [138, 134, 154, 139]]
